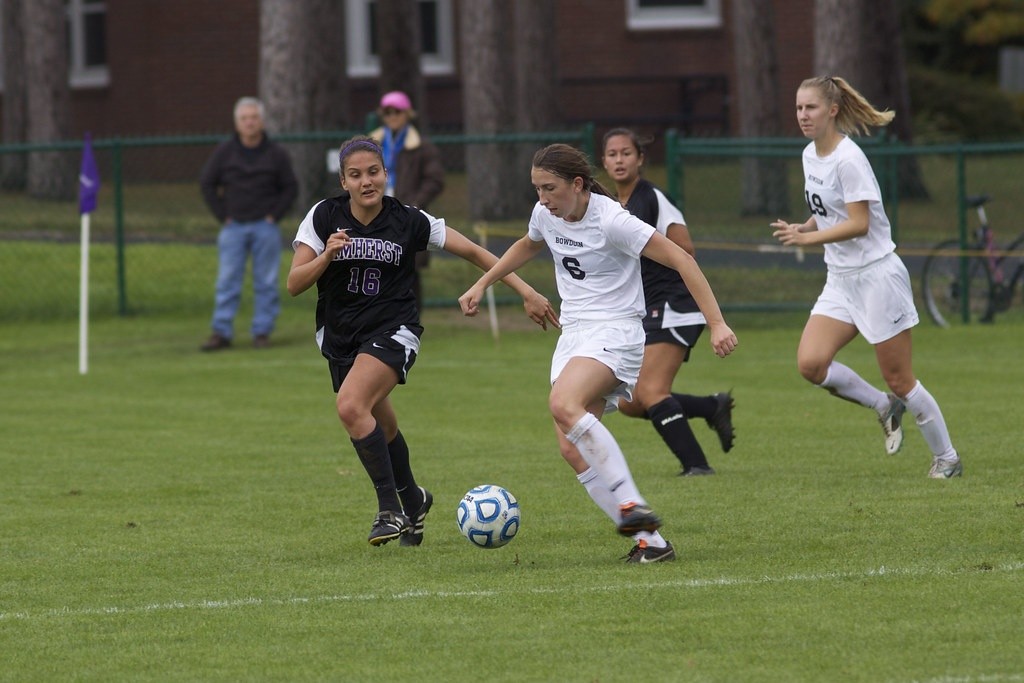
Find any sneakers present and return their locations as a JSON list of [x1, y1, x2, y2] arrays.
[[677, 467, 716, 476], [928, 452, 962, 479], [616, 502, 663, 535], [879, 394, 905, 454], [619, 539, 676, 566], [399, 486, 434, 547], [706, 391, 735, 453], [368, 511, 409, 547]]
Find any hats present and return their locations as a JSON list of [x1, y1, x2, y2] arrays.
[[380, 91, 411, 109]]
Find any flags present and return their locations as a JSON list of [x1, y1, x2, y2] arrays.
[[78, 134, 99, 211]]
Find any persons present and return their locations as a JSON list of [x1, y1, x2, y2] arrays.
[[599, 129, 736, 475], [197, 96, 300, 352], [287, 136, 560, 547], [767, 74, 962, 480], [457, 143, 738, 563], [369, 90, 446, 266]]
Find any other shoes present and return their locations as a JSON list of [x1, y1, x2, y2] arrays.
[[255, 334, 267, 348], [199, 335, 231, 349]]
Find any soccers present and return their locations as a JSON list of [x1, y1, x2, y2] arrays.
[[457, 484, 522, 550]]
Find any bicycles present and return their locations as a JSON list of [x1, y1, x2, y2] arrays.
[[919, 188, 1023, 332]]
